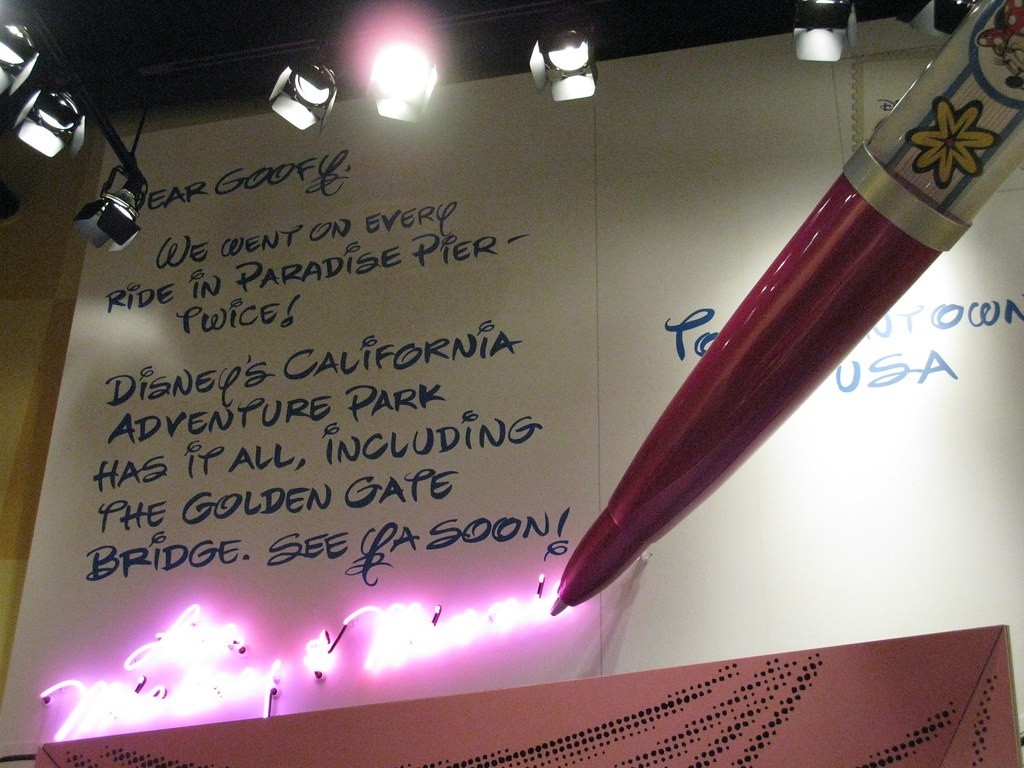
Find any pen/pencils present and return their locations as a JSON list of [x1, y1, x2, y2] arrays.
[[552, 0, 1024, 621]]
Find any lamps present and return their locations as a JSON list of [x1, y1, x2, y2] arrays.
[[269, 57, 336, 131], [527, 26, 598, 101], [789, 0, 860, 64], [0, 23, 46, 102], [74, 170, 151, 250], [15, 88, 92, 167]]
